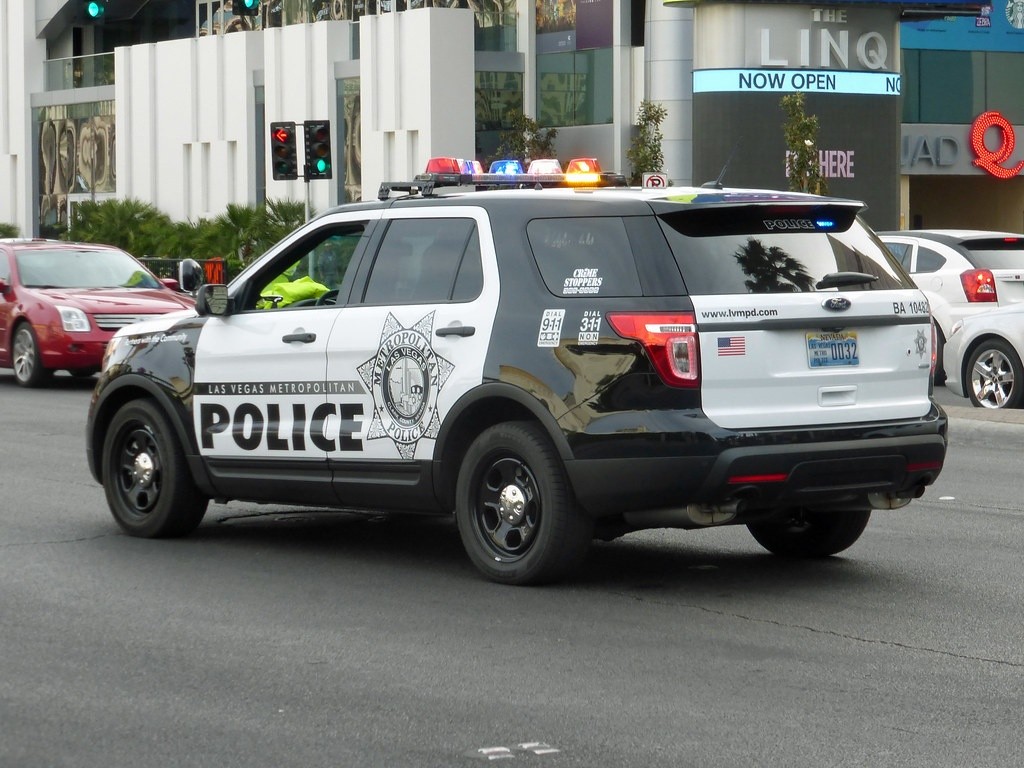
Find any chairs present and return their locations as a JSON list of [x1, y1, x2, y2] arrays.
[[412, 241, 477, 300]]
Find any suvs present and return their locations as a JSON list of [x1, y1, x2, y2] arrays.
[[86, 153, 949, 587]]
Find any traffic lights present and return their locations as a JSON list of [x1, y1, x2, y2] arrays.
[[271, 122, 298, 181], [304, 120, 333, 180], [83, 0, 107, 26], [232, 0, 259, 16]]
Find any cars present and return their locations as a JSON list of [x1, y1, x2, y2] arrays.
[[871, 230, 1024, 408], [0, 238, 200, 389]]
